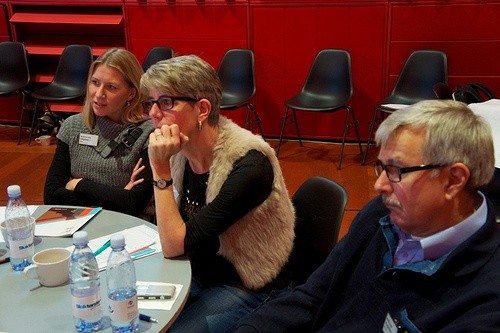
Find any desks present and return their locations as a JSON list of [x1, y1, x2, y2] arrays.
[[0, 205, 192, 333]]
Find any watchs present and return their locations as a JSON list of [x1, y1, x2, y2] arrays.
[[152, 178, 173, 189]]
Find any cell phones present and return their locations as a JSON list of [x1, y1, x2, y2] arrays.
[[135, 283, 177, 299]]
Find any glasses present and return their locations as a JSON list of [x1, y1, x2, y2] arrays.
[[142, 94, 196, 111], [373, 162, 437, 182]]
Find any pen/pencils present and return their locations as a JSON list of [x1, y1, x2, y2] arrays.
[[109, 304, 159, 323], [94, 240, 110, 256]]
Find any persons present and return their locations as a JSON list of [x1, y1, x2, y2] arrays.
[[232, 99, 500, 333], [43, 47, 156, 225], [139, 54, 297, 333]]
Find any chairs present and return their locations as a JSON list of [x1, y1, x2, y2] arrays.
[[216, 49, 266, 141], [275, 49, 364, 170], [29, 44, 93, 146], [141, 46, 174, 73], [269, 177, 348, 291], [362, 50, 448, 166], [0, 42, 30, 145]]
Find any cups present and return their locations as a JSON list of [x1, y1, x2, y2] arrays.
[[35, 136, 50, 145], [0, 217, 35, 247], [23, 247, 71, 287]]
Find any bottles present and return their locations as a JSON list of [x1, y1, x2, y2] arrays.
[[69, 231, 104, 333], [4, 184, 34, 270], [107, 233, 139, 333]]
